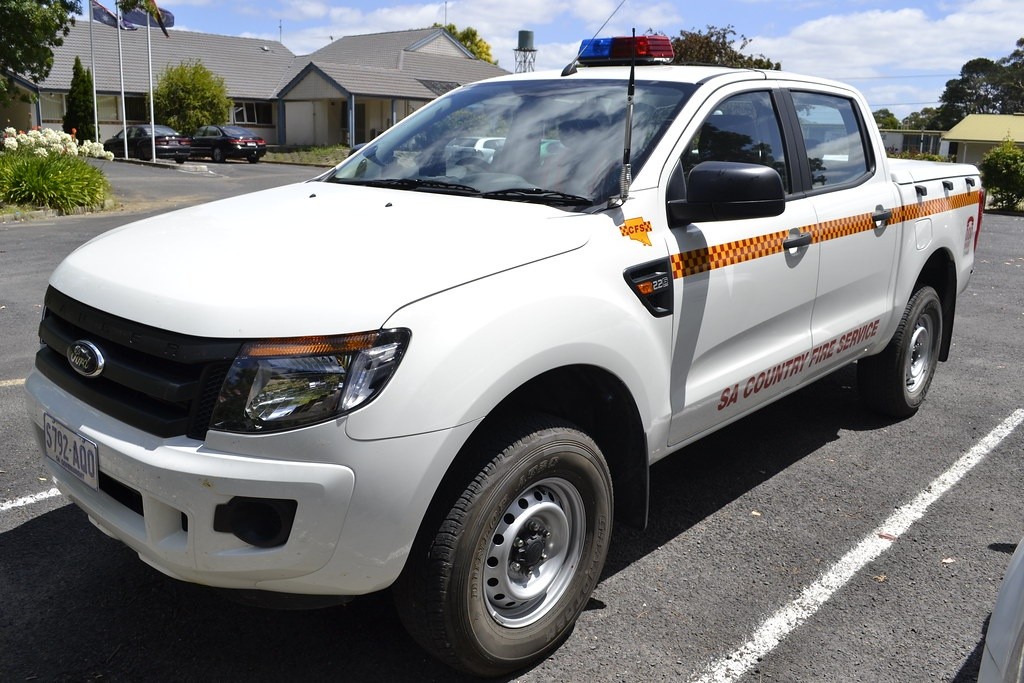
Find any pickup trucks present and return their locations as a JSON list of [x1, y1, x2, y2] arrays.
[[23, 33, 986, 677]]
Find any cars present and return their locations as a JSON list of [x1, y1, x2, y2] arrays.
[[189, 125, 267, 163], [104, 125, 191, 163]]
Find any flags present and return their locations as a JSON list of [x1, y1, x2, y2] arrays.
[[90, 0, 175, 39]]
